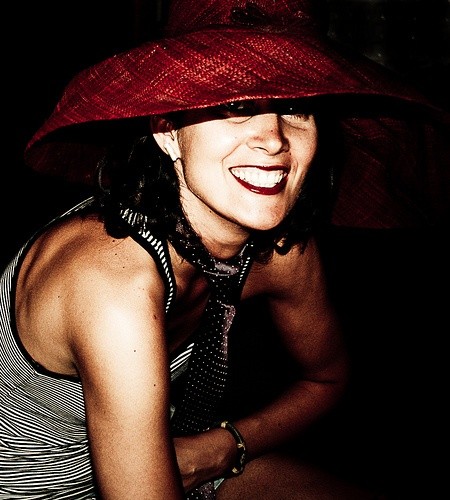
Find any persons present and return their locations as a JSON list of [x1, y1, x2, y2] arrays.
[[0, 2, 373, 500]]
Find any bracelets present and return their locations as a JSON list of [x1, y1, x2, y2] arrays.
[[213, 421, 250, 477]]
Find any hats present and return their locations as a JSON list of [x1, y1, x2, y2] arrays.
[[24, 0, 450, 229]]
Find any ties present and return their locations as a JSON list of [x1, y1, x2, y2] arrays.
[[168, 214, 256, 499]]
